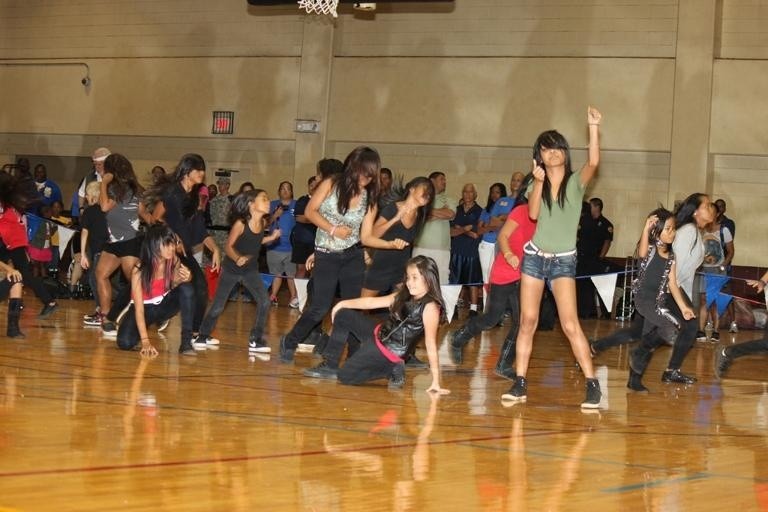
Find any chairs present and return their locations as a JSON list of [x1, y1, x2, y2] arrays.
[[387, 355, 429, 388], [269, 295, 299, 308], [39, 302, 58, 318], [279, 329, 329, 363], [180, 333, 220, 355], [729, 321, 739, 333], [574, 340, 603, 409], [502, 376, 528, 400], [303, 362, 340, 379], [83, 306, 170, 335], [713, 345, 731, 380], [696, 319, 720, 342], [248, 335, 272, 353], [661, 370, 698, 384], [453, 298, 511, 326]]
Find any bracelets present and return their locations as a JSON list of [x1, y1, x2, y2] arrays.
[[587, 123, 600, 126]]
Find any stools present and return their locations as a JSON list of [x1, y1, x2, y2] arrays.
[[314, 245, 332, 253], [522, 239, 578, 259]]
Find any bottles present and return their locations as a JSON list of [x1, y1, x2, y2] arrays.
[[216, 176, 231, 184]]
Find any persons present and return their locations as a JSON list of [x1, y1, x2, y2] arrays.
[[510, 411, 596, 512], [719, 425, 767, 512], [575, 192, 766, 391], [640, 426, 682, 512], [609, 418, 651, 512], [0, 146, 613, 394], [501, 104, 602, 408], [467, 430, 523, 512]]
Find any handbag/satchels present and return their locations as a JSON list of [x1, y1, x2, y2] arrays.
[[587, 123, 600, 126]]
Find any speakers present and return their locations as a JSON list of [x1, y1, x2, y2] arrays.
[[494, 337, 518, 381], [627, 328, 667, 393], [7, 298, 25, 338], [449, 324, 473, 363]]
